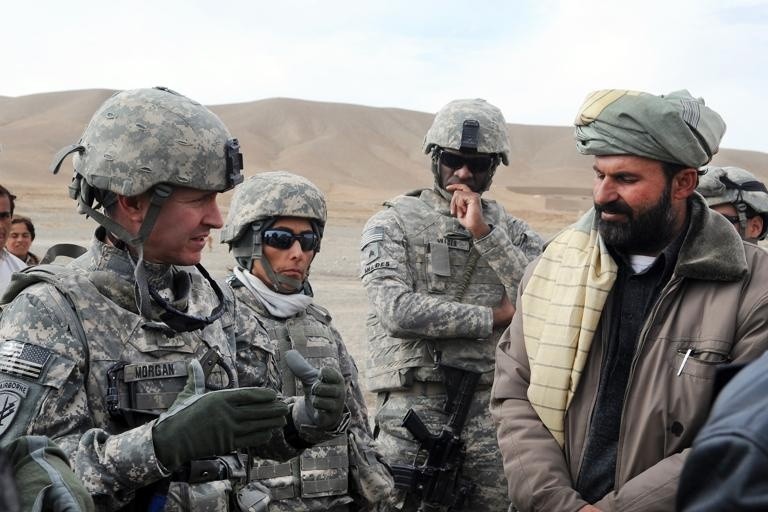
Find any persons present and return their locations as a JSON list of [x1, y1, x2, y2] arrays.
[[3, 214, 40, 266], [1, 88, 353, 512], [676, 346, 767, 511], [218, 170, 395, 512], [0, 184, 29, 303], [359, 99, 547, 512], [487, 88, 767, 511]]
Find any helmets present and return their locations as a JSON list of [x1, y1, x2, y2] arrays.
[[420, 98, 510, 167], [72, 88, 243, 195], [221, 172, 327, 247]]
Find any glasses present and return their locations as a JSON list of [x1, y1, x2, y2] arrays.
[[437, 152, 494, 172], [261, 227, 317, 252]]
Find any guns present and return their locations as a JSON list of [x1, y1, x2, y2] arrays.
[[387, 364, 483, 512]]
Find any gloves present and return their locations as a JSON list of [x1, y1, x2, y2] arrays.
[[285, 351, 344, 430], [151, 359, 287, 471]]
